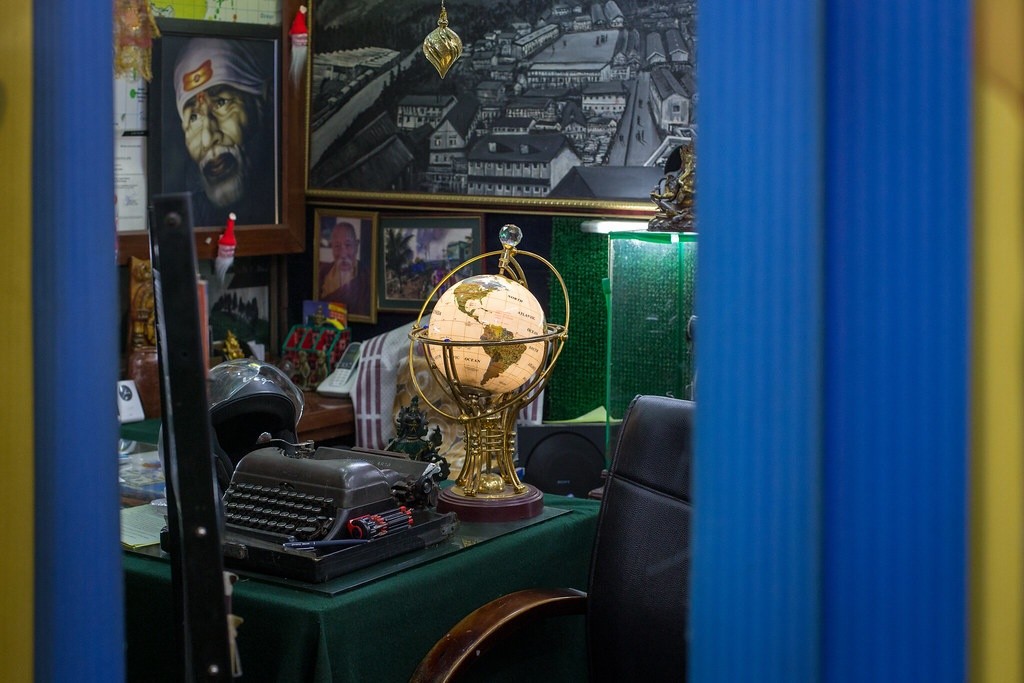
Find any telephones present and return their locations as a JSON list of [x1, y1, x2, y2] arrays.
[[316, 342, 363, 398]]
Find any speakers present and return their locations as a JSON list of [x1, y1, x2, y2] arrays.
[[517, 421, 621, 499]]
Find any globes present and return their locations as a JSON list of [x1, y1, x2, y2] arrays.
[[409, 220, 572, 526]]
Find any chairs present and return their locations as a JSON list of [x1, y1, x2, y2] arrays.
[[410, 394, 694, 683]]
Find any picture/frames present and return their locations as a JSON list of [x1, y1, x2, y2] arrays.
[[304, 0, 695, 219], [313, 209, 379, 324], [198, 255, 288, 369], [145, 16, 279, 228], [378, 213, 487, 314]]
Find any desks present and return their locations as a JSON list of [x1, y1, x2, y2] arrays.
[[119, 386, 355, 445], [119, 477, 602, 682]]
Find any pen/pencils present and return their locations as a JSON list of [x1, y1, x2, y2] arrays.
[[283, 538, 370, 551]]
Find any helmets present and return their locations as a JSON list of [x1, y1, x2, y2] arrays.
[[158, 358, 305, 495]]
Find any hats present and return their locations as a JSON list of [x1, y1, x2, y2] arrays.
[[173, 36, 276, 117]]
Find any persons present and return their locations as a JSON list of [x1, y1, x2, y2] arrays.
[[318, 224, 370, 315], [216, 214, 238, 280]]
